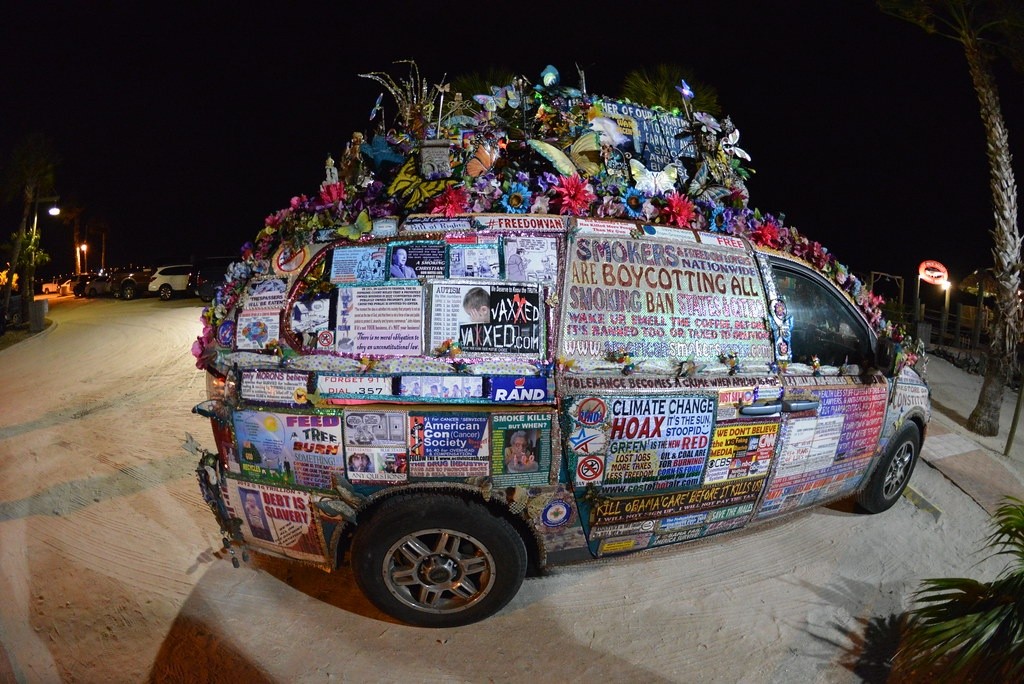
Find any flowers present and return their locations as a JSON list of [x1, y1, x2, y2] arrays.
[[187, 55, 929, 383]]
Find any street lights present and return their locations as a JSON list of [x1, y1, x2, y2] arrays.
[[29, 194, 62, 302]]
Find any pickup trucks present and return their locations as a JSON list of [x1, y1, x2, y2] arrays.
[[42, 277, 64, 294]]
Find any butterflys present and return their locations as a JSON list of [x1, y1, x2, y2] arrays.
[[723, 128, 751, 161], [534, 361, 555, 377], [545, 291, 559, 308], [304, 260, 339, 303], [675, 79, 694, 99], [687, 160, 732, 203], [181, 432, 201, 456], [679, 352, 707, 381], [191, 363, 247, 431], [434, 338, 475, 375], [204, 460, 221, 486], [305, 388, 321, 405], [473, 85, 516, 111], [433, 83, 450, 93], [767, 313, 793, 344], [464, 476, 493, 502], [893, 414, 903, 431], [333, 207, 373, 242], [534, 64, 582, 100], [314, 472, 374, 526], [387, 153, 464, 209], [506, 87, 535, 110], [508, 485, 553, 523], [558, 355, 575, 373], [629, 158, 678, 198], [526, 131, 601, 179], [702, 139, 728, 179], [464, 134, 500, 177], [418, 277, 427, 286]]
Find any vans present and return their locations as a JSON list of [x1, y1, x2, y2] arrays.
[[198, 208, 935, 629]]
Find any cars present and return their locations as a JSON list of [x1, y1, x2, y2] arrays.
[[70, 275, 88, 290], [105, 263, 158, 299], [148, 263, 197, 301], [60, 280, 76, 295], [187, 256, 249, 302], [86, 275, 113, 296], [74, 276, 102, 296]]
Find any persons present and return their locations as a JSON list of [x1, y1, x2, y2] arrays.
[[505, 430, 533, 466], [348, 452, 371, 473], [390, 247, 417, 278], [243, 493, 263, 529], [462, 287, 490, 322]]
